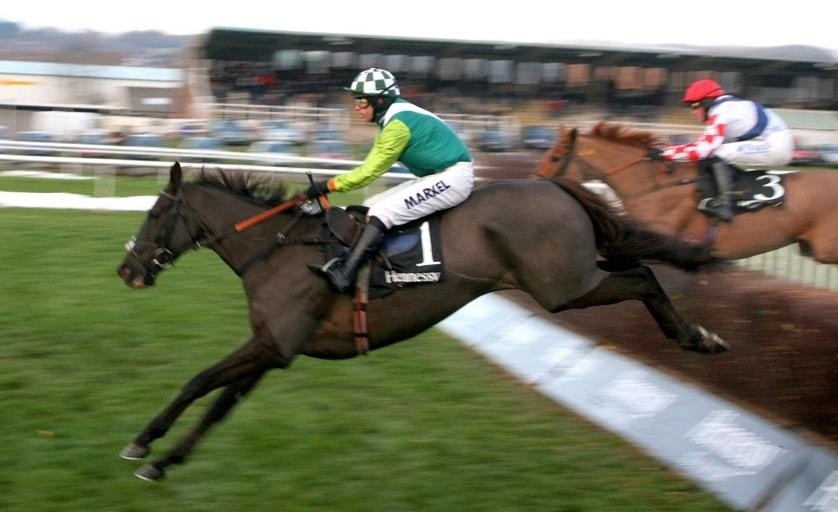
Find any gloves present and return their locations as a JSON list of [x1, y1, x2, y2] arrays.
[[645, 148, 665, 161], [305, 179, 331, 199]]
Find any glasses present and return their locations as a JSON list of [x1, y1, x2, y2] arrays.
[[351, 95, 374, 110], [687, 101, 702, 109]]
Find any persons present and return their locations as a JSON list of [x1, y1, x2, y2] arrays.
[[645, 79, 794, 221], [300, 66, 475, 292]]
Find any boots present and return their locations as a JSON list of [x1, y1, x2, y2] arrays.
[[307, 222, 386, 295], [696, 161, 735, 222]]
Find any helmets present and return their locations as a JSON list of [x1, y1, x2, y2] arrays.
[[680, 79, 725, 105], [341, 67, 402, 98]]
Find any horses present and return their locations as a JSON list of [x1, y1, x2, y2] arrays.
[[524, 119, 838, 266], [116, 157, 731, 484]]
